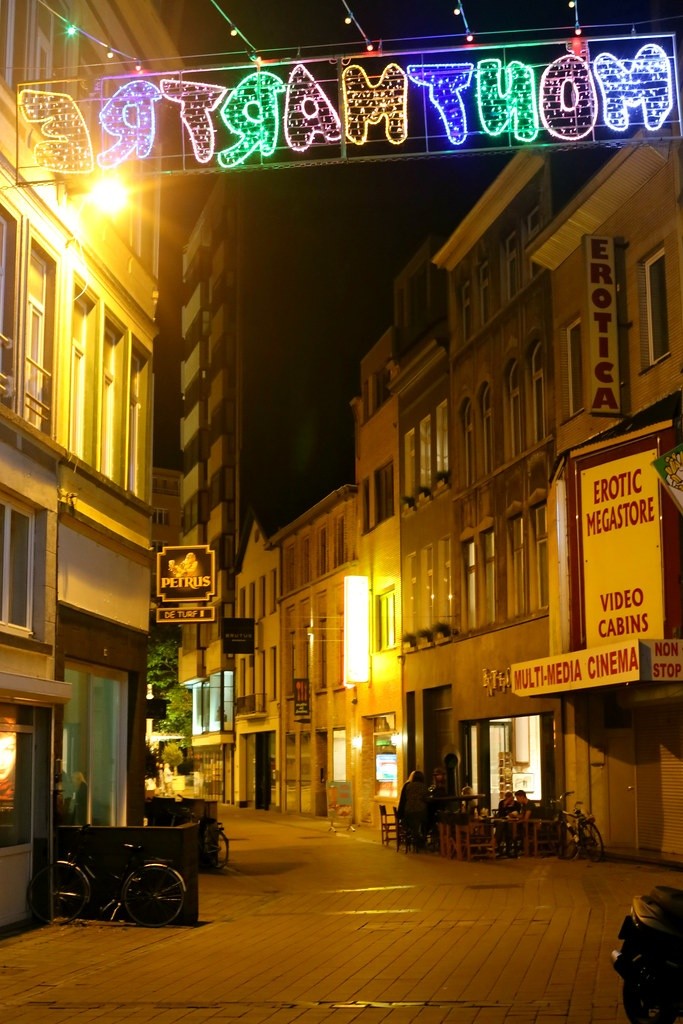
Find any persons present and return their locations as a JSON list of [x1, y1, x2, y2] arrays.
[[511, 790, 540, 856], [396, 780, 412, 836], [71, 770, 88, 826], [403, 771, 432, 850], [461, 786, 476, 814], [429, 769, 447, 812], [495, 791, 522, 855]]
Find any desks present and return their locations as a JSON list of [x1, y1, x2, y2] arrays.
[[489, 817, 541, 858]]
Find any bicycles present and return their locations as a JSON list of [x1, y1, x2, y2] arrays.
[[26, 824, 187, 928], [197, 815, 229, 871], [550, 790, 604, 863]]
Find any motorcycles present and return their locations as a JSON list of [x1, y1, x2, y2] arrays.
[[611, 885, 683, 1024]]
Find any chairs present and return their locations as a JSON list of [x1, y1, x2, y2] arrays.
[[379, 805, 563, 864]]
